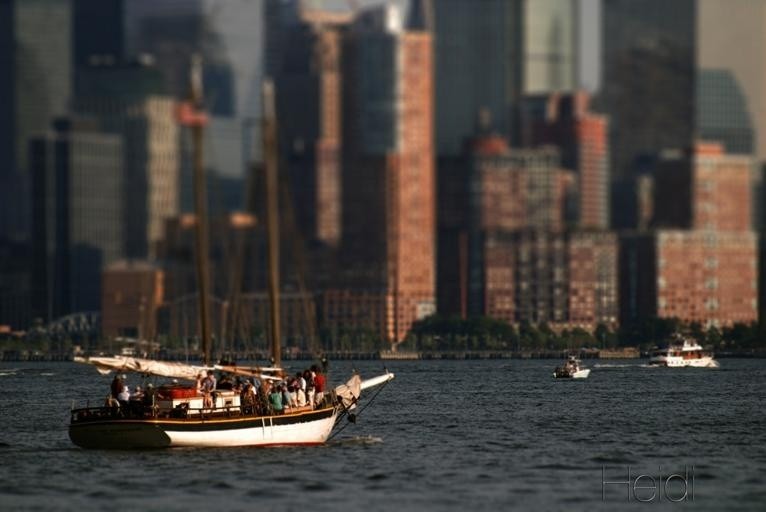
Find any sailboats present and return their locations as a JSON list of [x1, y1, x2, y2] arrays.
[[69, 80, 392, 448]]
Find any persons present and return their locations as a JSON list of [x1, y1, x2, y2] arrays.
[[196, 364, 325, 423], [567, 355, 580, 371], [111, 375, 164, 415]]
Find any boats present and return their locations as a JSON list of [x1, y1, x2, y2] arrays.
[[553, 358, 590, 379], [649, 337, 718, 367]]
